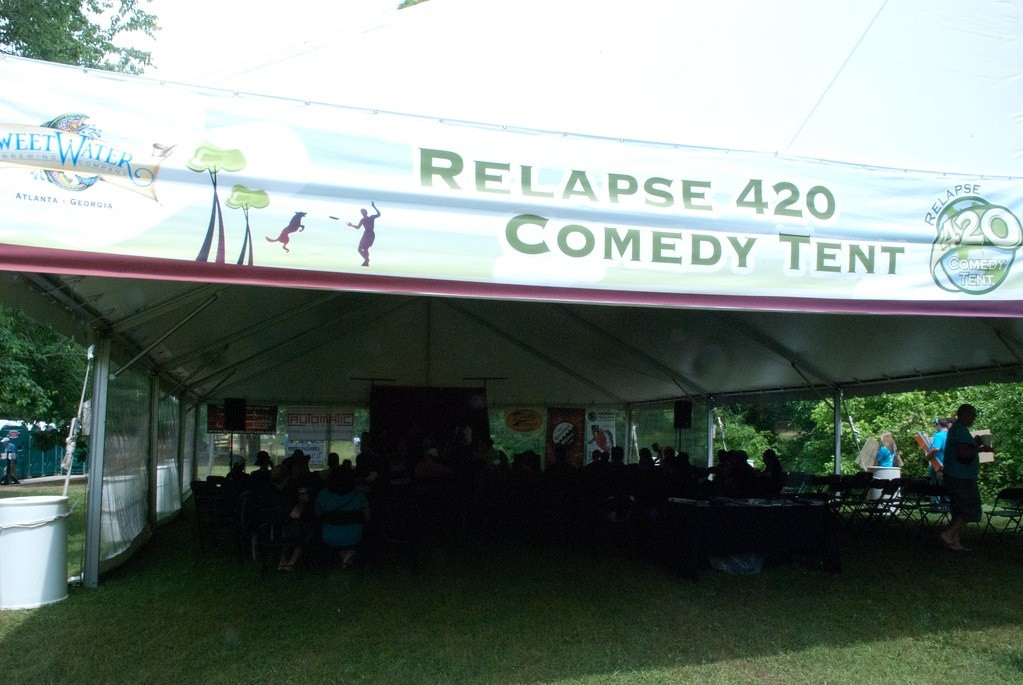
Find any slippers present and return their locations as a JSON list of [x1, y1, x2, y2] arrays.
[[936, 534, 955, 547], [948, 545, 973, 554]]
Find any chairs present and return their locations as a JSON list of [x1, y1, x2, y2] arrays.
[[773, 471, 952, 535], [189, 473, 423, 576], [984, 487, 1023, 538]]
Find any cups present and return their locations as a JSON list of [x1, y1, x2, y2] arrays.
[[980, 435, 992, 450]]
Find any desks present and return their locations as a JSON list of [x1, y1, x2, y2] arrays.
[[668, 498, 843, 582]]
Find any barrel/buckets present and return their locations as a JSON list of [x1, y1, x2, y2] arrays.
[[101, 475, 143, 541], [867, 466, 902, 516], [746, 459, 755, 469], [967, 429, 994, 463], [651, 457, 661, 466], [165, 459, 179, 494], [141, 465, 171, 512], [0, 495, 72, 609], [182, 459, 194, 493]]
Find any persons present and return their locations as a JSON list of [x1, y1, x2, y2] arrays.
[[213, 426, 578, 572], [925, 418, 955, 511], [580, 442, 785, 545], [936, 404, 995, 553], [1, 437, 17, 484], [876, 434, 904, 467]]
